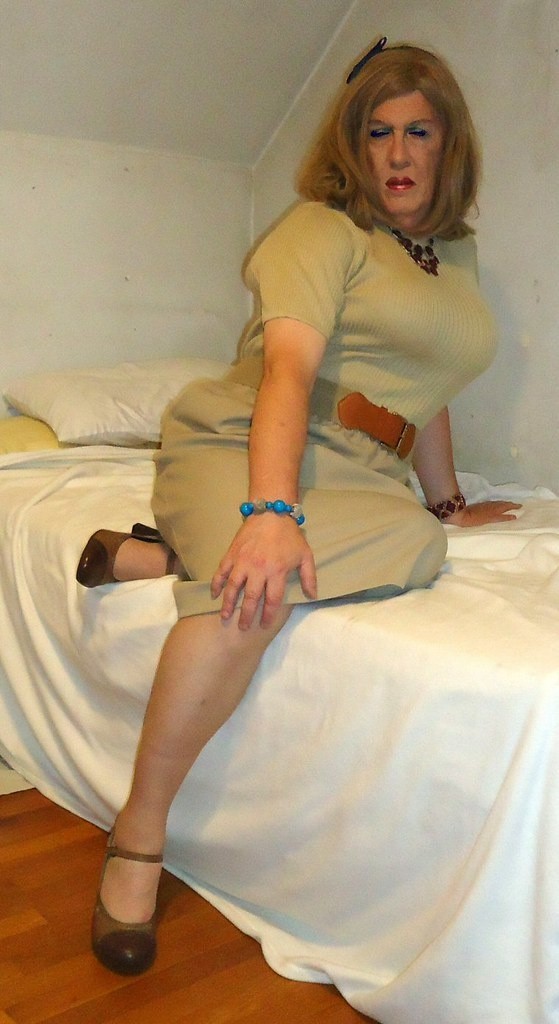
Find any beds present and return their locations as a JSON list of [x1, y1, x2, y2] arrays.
[[1, 389, 559, 1024]]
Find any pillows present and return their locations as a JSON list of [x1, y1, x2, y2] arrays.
[[2, 355, 246, 445]]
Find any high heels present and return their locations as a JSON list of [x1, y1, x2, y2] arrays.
[[76, 523, 175, 587], [89, 827, 163, 974]]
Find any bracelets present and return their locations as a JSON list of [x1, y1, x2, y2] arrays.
[[428, 493, 466, 520], [240, 501, 305, 526]]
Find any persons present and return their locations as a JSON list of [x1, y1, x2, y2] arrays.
[[76, 37, 522, 974]]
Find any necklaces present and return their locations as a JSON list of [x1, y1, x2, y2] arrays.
[[387, 223, 440, 276]]
